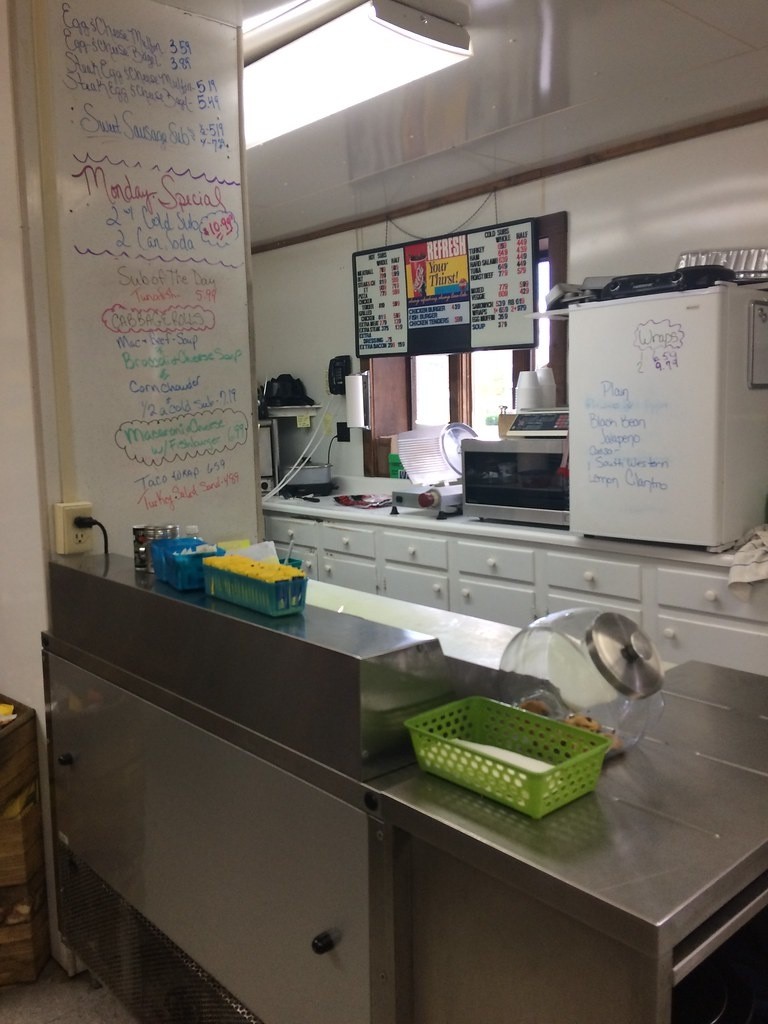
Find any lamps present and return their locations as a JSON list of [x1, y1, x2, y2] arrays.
[[243, 0, 474, 148]]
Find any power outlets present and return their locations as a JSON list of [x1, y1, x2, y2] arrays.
[[54, 502, 94, 554]]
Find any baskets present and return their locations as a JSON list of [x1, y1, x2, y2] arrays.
[[405, 695, 614, 823]]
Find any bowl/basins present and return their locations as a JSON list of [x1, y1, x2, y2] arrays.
[[514, 367, 557, 410]]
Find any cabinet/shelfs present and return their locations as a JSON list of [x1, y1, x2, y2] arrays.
[[265, 503, 768, 677]]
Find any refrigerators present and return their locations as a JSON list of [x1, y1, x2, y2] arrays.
[[567, 280, 767, 551]]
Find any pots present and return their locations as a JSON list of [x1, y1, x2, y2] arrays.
[[282, 456, 333, 498]]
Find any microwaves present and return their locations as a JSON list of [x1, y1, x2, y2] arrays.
[[460, 439, 570, 526]]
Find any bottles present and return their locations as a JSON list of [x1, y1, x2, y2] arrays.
[[133, 524, 204, 573], [498, 606, 668, 760]]
